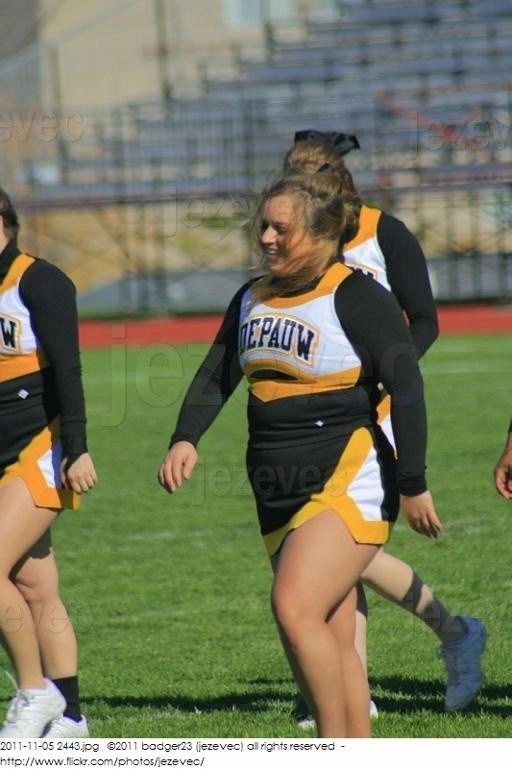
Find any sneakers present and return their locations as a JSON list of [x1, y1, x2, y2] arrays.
[[3, 676, 65, 741], [441, 614, 485, 711], [299, 697, 379, 729], [45, 715, 90, 736]]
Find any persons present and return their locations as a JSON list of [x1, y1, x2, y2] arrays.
[[492, 417, 512, 501], [0, 184, 98, 740], [156, 172, 444, 739], [279, 139, 488, 731]]
